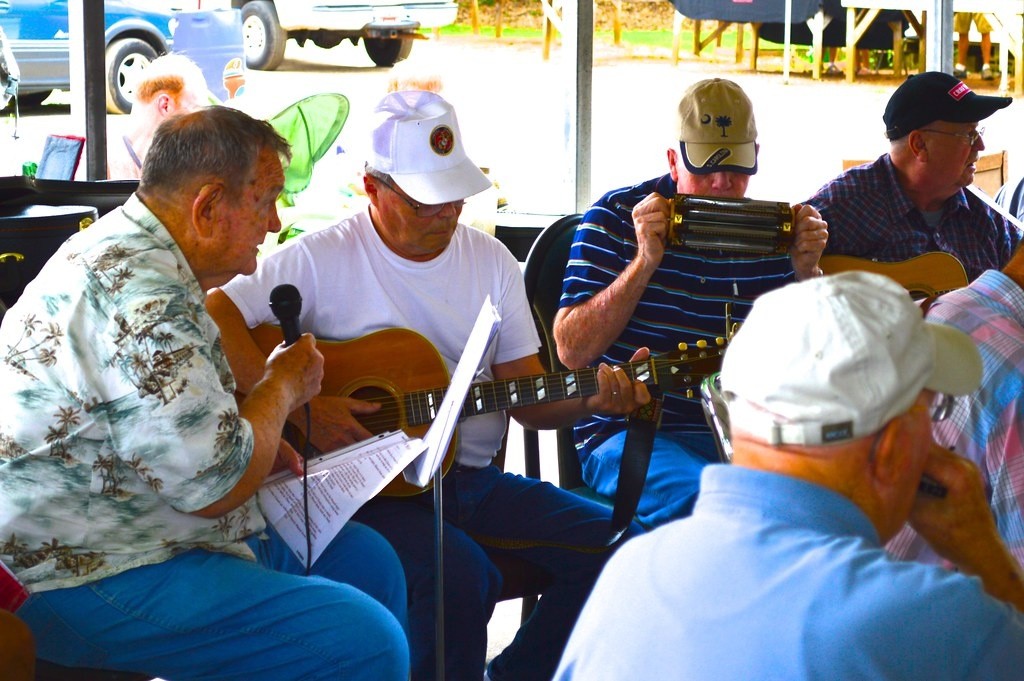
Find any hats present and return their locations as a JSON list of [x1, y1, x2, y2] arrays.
[[720, 270, 983, 448], [362, 90, 492, 205], [676, 78, 758, 174], [883, 71, 1013, 142]]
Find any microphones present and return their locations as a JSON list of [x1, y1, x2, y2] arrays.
[[269, 283, 303, 347]]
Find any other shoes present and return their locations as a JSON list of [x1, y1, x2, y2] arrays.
[[981, 68, 995, 80], [953, 68, 967, 78], [484, 654, 509, 681]]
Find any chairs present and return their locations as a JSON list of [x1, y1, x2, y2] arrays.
[[523, 213, 583, 489]]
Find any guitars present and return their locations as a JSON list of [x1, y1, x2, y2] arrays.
[[799, 250, 970, 320], [233, 320, 747, 498]]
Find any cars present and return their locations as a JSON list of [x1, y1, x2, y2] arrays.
[[180, 0, 458, 72], [0, 0, 179, 115]]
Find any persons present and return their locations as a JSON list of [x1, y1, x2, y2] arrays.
[[0, 104, 410, 681], [115, 55, 1024, 681]]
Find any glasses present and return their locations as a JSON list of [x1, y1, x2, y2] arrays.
[[921, 126, 985, 146], [914, 394, 957, 422], [370, 174, 467, 217]]
